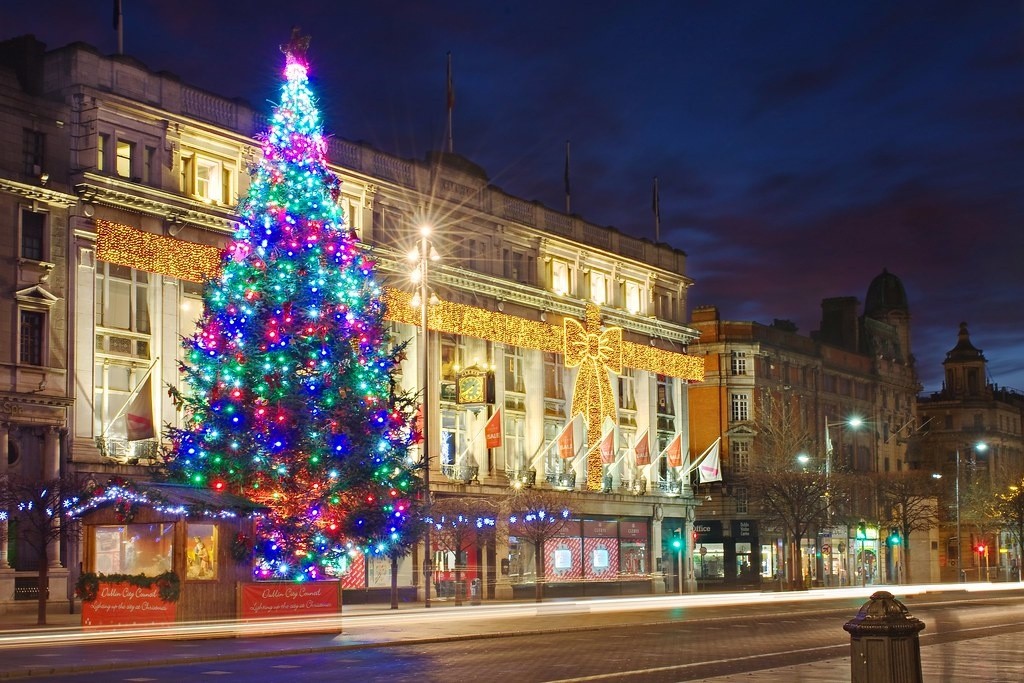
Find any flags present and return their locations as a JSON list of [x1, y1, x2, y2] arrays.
[[558, 421, 574, 458], [698, 441, 722, 484], [666, 435, 682, 467], [601, 428, 615, 464], [635, 431, 651, 466], [125, 372, 154, 441], [484, 408, 502, 449]]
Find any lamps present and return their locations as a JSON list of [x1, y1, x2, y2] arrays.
[[407, 238, 442, 309]]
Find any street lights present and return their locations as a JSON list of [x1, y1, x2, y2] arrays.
[[407, 223, 441, 609], [824, 416, 864, 586], [955, 444, 988, 583]]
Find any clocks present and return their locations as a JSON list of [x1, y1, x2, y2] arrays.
[[455, 371, 496, 407]]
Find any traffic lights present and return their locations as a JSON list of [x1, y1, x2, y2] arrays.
[[691, 532, 697, 549], [977, 543, 984, 552], [892, 528, 899, 544], [673, 534, 680, 550]]
